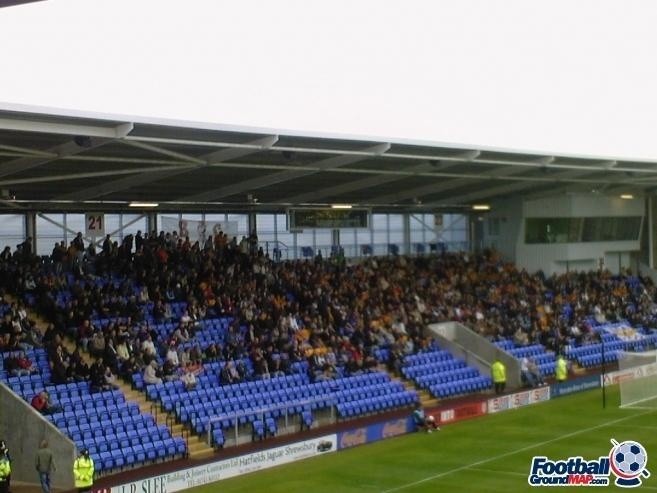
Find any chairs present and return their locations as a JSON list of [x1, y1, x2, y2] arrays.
[[0, 301, 657, 474]]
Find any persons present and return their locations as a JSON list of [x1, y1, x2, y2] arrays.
[[411, 400, 441, 433], [0, 439, 14, 493], [0, 229, 656, 414], [73, 448, 95, 493], [35, 439, 56, 493]]
